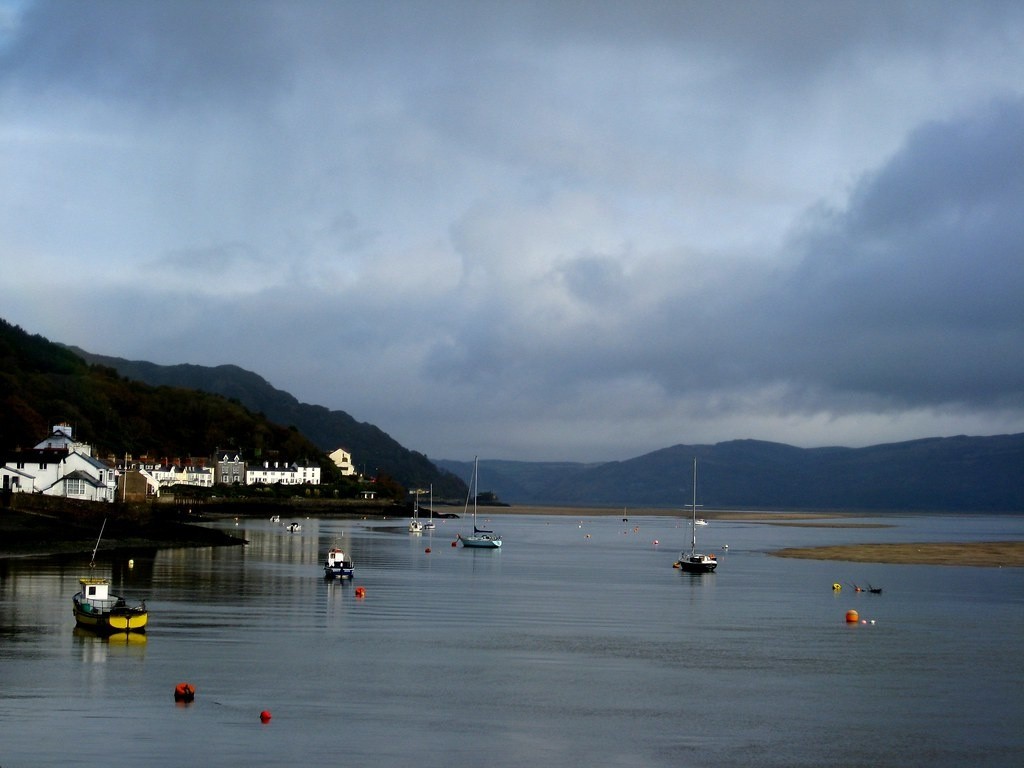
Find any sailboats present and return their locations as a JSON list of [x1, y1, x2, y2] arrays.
[[458, 456, 503, 547], [678, 454, 718, 574], [423, 483, 436, 529], [409, 490, 423, 532]]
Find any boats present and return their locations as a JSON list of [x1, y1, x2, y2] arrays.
[[695, 518, 710, 525], [287, 523, 302, 531], [74, 621, 146, 643], [270, 516, 280, 523], [72, 518, 149, 630], [324, 528, 354, 580]]
